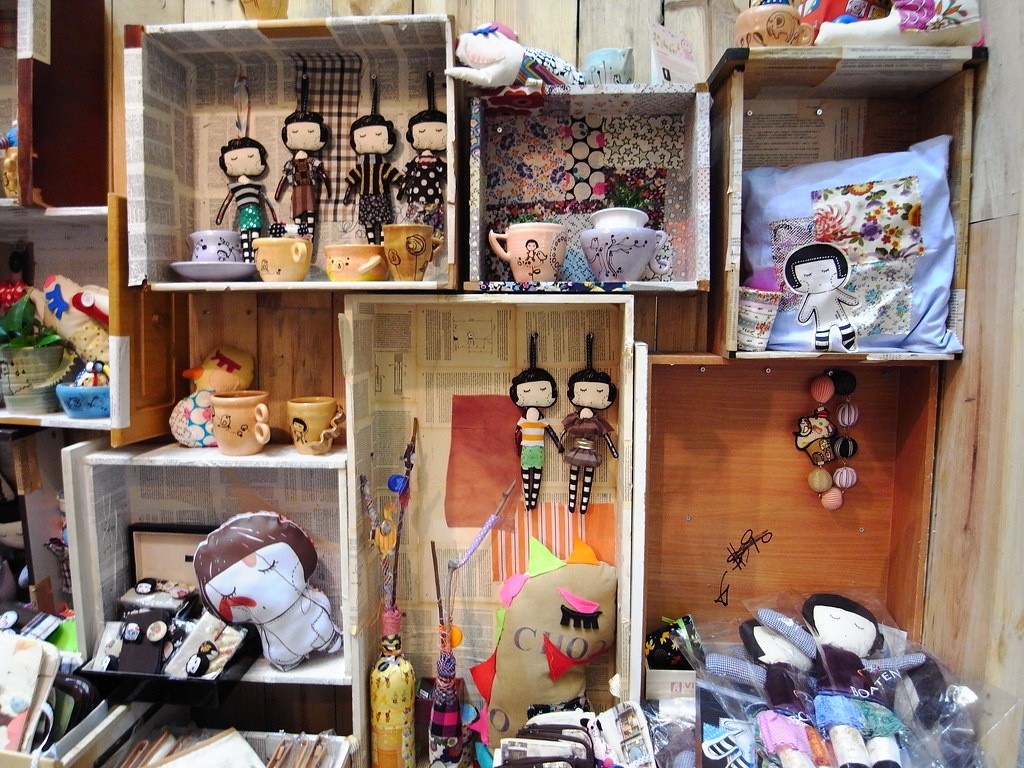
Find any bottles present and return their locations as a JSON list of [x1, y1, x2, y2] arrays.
[[370, 612, 416, 768], [3, 146, 18, 198], [428, 651, 463, 768]]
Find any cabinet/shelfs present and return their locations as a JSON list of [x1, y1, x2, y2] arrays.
[[0, 0, 989, 768]]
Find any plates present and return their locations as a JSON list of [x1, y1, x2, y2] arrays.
[[170, 261, 255, 280]]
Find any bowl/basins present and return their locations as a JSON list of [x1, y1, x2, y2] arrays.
[[56, 382, 109, 418], [324, 245, 390, 281]]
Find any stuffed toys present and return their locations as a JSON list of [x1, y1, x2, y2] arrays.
[[187, 639, 220, 676], [0, 275, 109, 388], [136, 574, 192, 600], [466, 534, 617, 757], [168, 342, 254, 446]]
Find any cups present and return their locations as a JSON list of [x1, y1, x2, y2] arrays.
[[288, 395, 337, 453], [580, 207, 667, 282], [580, 46, 635, 83], [734, 4, 814, 47], [489, 222, 567, 282], [382, 225, 441, 282], [251, 237, 313, 281], [645, 615, 706, 669], [210, 391, 270, 456], [738, 286, 780, 351], [189, 230, 244, 262]]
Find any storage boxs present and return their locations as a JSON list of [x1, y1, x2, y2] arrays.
[[73, 522, 263, 708]]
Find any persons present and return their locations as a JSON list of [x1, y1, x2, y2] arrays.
[[194, 509, 343, 671], [0, 498, 31, 603], [396, 110, 448, 249], [44, 487, 74, 616], [215, 136, 277, 263], [273, 108, 332, 250], [341, 114, 406, 247], [507, 366, 565, 511], [705, 591, 931, 768], [558, 369, 619, 514]]
